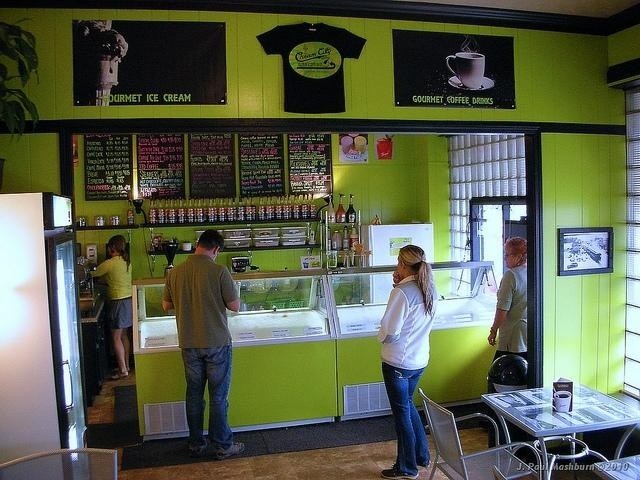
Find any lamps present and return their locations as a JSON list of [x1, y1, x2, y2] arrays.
[[318, 194, 330, 219], [133, 198, 148, 224]]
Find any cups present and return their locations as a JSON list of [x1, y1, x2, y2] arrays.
[[446, 52, 485, 89], [552, 390, 572, 412], [340, 133, 393, 160]]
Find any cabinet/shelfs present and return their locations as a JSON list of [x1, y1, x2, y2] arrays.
[[136, 267, 337, 443], [329, 260, 504, 453], [327, 220, 367, 259]]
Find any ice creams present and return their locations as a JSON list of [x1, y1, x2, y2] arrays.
[[72, 19, 128, 105]]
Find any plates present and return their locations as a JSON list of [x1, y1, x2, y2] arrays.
[[446, 75, 494, 92]]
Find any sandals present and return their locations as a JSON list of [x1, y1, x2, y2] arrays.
[[111, 371, 129, 379]]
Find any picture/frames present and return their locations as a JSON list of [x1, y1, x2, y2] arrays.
[[557, 227, 614, 275]]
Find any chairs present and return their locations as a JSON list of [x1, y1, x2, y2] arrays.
[[416, 386, 543, 480], [589, 455, 640, 480], [1, 446, 118, 480]]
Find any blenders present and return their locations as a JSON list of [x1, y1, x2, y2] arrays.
[[230, 254, 252, 274]]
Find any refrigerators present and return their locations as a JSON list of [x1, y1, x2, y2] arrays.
[[0, 192, 91, 480]]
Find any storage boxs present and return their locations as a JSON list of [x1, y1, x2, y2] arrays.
[[195, 227, 308, 250]]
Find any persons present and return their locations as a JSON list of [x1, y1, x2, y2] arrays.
[[162, 228, 245, 460], [89, 235, 133, 380], [486, 237, 527, 360], [378, 243, 435, 479]]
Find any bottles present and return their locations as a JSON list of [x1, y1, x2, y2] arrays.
[[327, 194, 360, 269], [127, 199, 134, 226], [148, 192, 317, 225], [307, 224, 317, 245]]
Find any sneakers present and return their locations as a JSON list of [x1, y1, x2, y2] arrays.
[[381, 469, 419, 480], [189, 436, 210, 457], [216, 442, 245, 460]]
